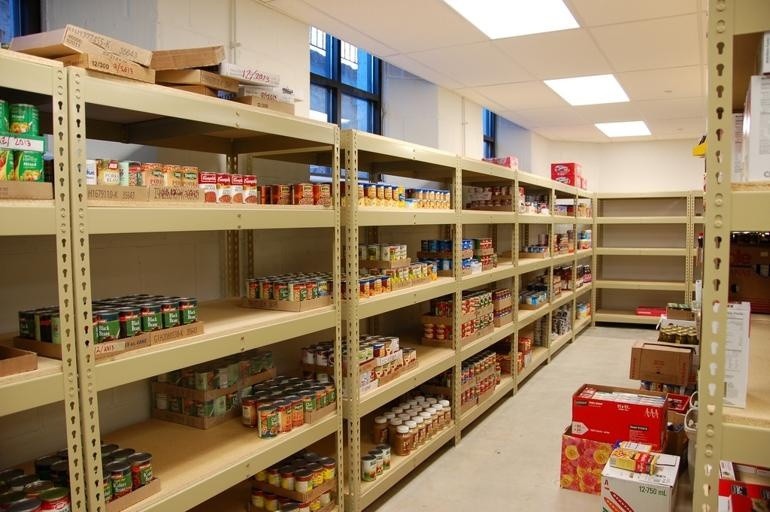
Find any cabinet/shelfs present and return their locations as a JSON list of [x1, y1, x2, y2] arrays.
[[691, 1, 770, 511], [246, 127, 597, 511], [593, 191, 690, 326], [64, 64, 345, 512], [0, 44, 90, 512]]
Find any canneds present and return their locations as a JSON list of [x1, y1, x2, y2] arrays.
[[241, 376, 336, 439], [494, 287, 513, 328], [658, 325, 700, 344], [406, 351, 497, 405], [242, 238, 498, 301], [18, 293, 200, 343], [360, 444, 391, 480], [0, 102, 44, 182], [2, 437, 155, 511], [256, 182, 451, 208], [250, 448, 336, 512]]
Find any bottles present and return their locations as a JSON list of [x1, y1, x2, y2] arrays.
[[495, 362, 501, 384], [549, 265, 592, 291], [668, 398, 674, 408]]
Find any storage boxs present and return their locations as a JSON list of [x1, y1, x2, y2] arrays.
[[233, 95, 297, 115], [155, 68, 239, 93], [51, 50, 154, 85], [237, 86, 291, 103], [9, 31, 104, 60], [149, 45, 226, 71], [218, 64, 282, 85], [557, 302, 699, 511], [216, 84, 294, 104], [64, 25, 154, 69]]
